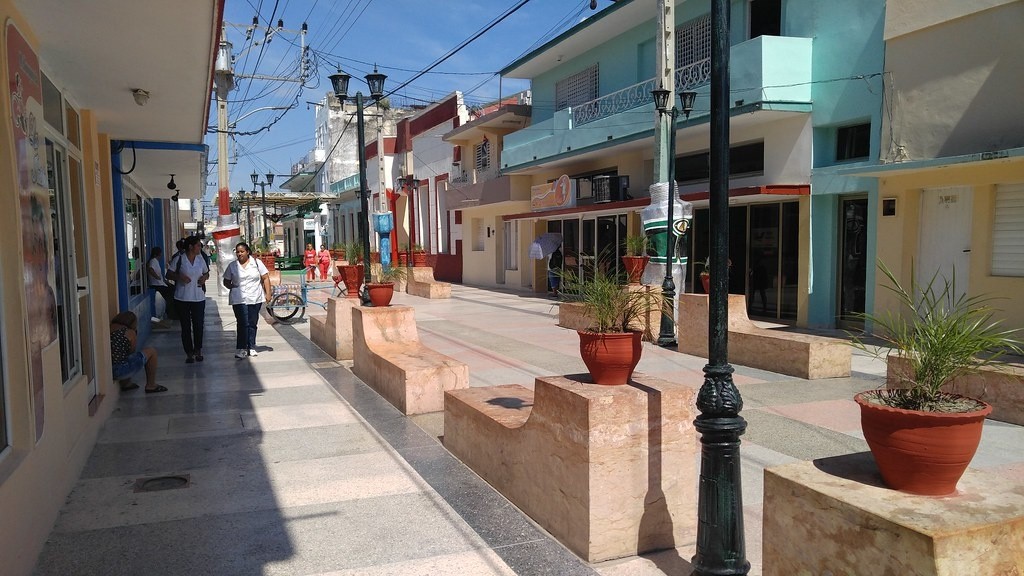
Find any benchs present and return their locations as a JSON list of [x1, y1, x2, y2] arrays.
[[274, 257, 301, 269]]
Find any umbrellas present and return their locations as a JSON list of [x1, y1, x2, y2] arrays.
[[528, 234, 562, 260]]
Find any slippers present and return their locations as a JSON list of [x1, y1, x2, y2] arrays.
[[144, 384, 168, 393], [120, 383, 138, 390]]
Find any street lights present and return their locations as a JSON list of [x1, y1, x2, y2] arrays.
[[238, 185, 257, 247], [250, 169, 275, 246], [327, 62, 389, 307], [649, 78, 698, 348]]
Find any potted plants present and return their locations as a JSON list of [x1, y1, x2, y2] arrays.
[[329, 235, 428, 306], [835, 255, 1024, 493], [530, 233, 681, 384], [693, 257, 713, 293]]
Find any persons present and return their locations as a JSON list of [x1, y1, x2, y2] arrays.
[[752, 248, 771, 309], [546, 244, 564, 298], [274, 248, 289, 258], [302, 243, 318, 283], [319, 245, 331, 283], [130, 246, 141, 295], [146, 246, 175, 301], [165, 235, 212, 364], [222, 242, 273, 360], [109, 310, 168, 392]]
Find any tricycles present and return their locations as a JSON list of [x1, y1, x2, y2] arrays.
[[265, 264, 365, 325]]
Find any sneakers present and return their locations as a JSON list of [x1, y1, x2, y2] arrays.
[[235, 350, 248, 359], [248, 348, 257, 357]]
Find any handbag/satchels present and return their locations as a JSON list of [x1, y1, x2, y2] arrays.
[[314, 267, 321, 276], [327, 267, 333, 276]]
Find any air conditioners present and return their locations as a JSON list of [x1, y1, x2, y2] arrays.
[[592, 174, 628, 202]]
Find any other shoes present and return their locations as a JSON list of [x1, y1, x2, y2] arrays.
[[194, 350, 203, 362], [185, 357, 194, 363]]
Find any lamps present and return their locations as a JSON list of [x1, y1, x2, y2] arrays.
[[167, 174, 177, 189], [172, 190, 180, 202], [133, 88, 150, 106]]
